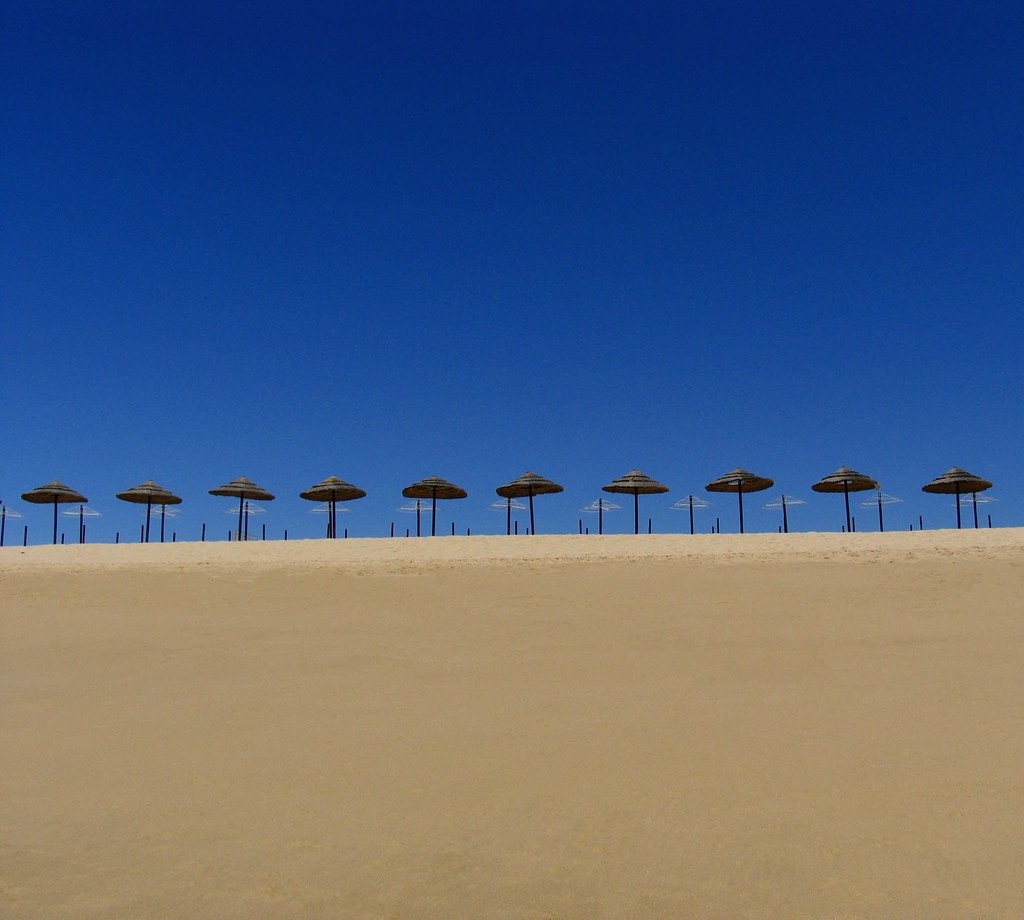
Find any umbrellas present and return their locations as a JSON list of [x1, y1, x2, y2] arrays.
[[761, 492, 807, 533], [402, 475, 468, 537], [668, 494, 710, 535], [226, 500, 267, 541], [312, 502, 349, 538], [21, 480, 88, 544], [860, 491, 904, 532], [580, 497, 620, 535], [496, 471, 564, 535], [208, 476, 275, 541], [61, 504, 101, 543], [705, 468, 775, 533], [811, 466, 880, 532], [150, 504, 181, 543], [299, 476, 368, 538], [0, 504, 23, 546], [116, 480, 182, 543], [489, 497, 527, 535], [602, 469, 671, 534], [951, 492, 1001, 529], [922, 466, 993, 529], [398, 499, 441, 537]]
